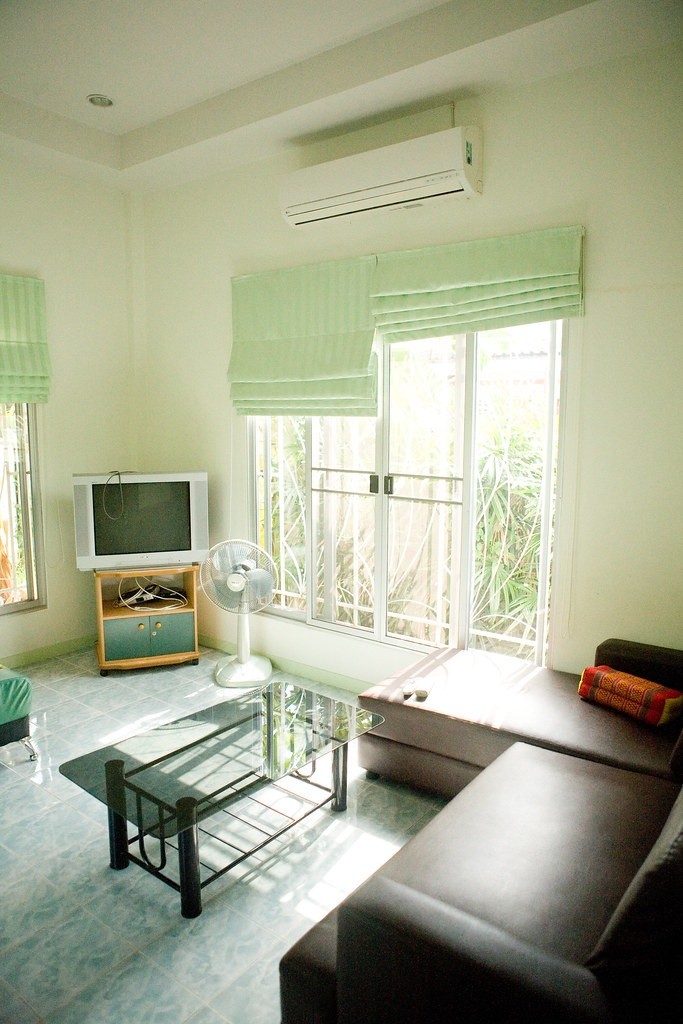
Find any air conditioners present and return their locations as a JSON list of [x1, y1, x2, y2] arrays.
[[283, 128, 482, 227]]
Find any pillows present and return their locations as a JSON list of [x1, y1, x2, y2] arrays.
[[578, 666, 683, 726]]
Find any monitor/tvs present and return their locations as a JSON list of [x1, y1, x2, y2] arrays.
[[71, 472, 208, 571]]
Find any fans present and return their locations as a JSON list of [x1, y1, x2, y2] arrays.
[[199, 540, 279, 688]]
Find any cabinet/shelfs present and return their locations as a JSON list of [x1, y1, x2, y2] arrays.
[[93, 566, 202, 677]]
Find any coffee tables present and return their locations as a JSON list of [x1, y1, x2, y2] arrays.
[[57, 682, 384, 919]]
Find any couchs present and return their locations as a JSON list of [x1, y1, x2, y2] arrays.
[[279, 638, 683, 1023], [0, 665, 39, 761]]
